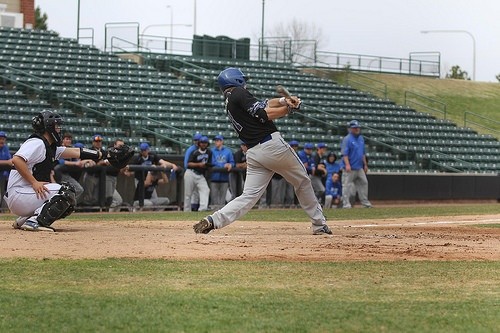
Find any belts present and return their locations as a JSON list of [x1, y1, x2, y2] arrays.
[[261, 135, 272, 144], [5, 192, 8, 197]]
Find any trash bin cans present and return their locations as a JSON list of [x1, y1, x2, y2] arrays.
[[235, 38, 250, 61], [191, 34, 202, 55], [216, 36, 235, 58], [202, 34, 216, 56]]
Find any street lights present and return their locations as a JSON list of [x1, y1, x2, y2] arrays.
[[141, 24, 192, 51], [420, 29, 476, 81], [166, 5, 173, 53]]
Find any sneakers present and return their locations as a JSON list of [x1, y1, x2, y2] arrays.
[[13, 221, 20, 230], [192, 216, 214, 234], [314, 226, 332, 236], [21, 221, 54, 233]]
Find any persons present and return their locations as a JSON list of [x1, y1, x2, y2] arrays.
[[0, 109, 375, 232], [193, 67, 335, 234]]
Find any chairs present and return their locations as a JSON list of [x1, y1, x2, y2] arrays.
[[0, 27, 500, 174]]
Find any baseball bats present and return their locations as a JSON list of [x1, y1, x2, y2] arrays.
[[277, 85, 298, 107]]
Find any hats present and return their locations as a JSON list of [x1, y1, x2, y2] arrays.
[[213, 135, 224, 141], [305, 144, 314, 149], [0, 131, 7, 138], [193, 135, 202, 142], [350, 121, 362, 128], [93, 135, 103, 142], [140, 143, 148, 150], [317, 143, 327, 148], [74, 143, 84, 148], [290, 141, 298, 147]]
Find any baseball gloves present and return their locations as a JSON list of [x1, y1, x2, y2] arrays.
[[101, 144, 135, 168]]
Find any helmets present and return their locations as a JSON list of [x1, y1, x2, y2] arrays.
[[198, 138, 210, 146], [32, 111, 63, 145], [217, 68, 249, 89]]
